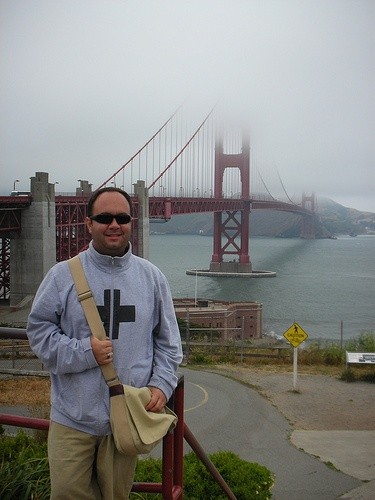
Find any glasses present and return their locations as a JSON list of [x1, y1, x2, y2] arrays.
[[91, 212, 133, 225]]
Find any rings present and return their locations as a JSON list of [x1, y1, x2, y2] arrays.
[[106, 353, 110, 359]]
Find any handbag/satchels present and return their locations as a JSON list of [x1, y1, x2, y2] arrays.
[[108, 384, 178, 456]]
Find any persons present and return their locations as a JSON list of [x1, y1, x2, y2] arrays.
[[26, 186, 184, 500]]
